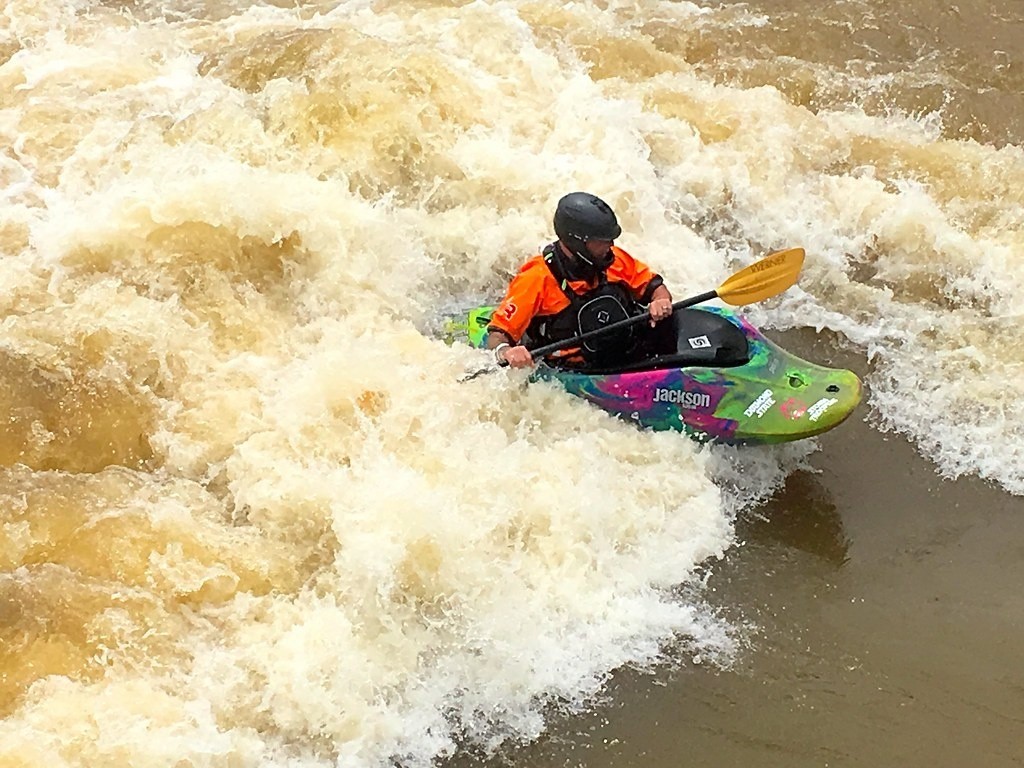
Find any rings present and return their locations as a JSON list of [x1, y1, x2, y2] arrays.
[[663, 306, 668, 309]]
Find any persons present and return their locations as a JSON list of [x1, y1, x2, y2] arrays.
[[480, 191, 673, 371]]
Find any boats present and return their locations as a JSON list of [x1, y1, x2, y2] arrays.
[[440, 302, 865, 448]]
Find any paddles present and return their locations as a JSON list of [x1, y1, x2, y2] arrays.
[[456, 247, 805, 384]]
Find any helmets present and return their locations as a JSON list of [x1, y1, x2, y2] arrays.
[[553, 192, 621, 272]]
